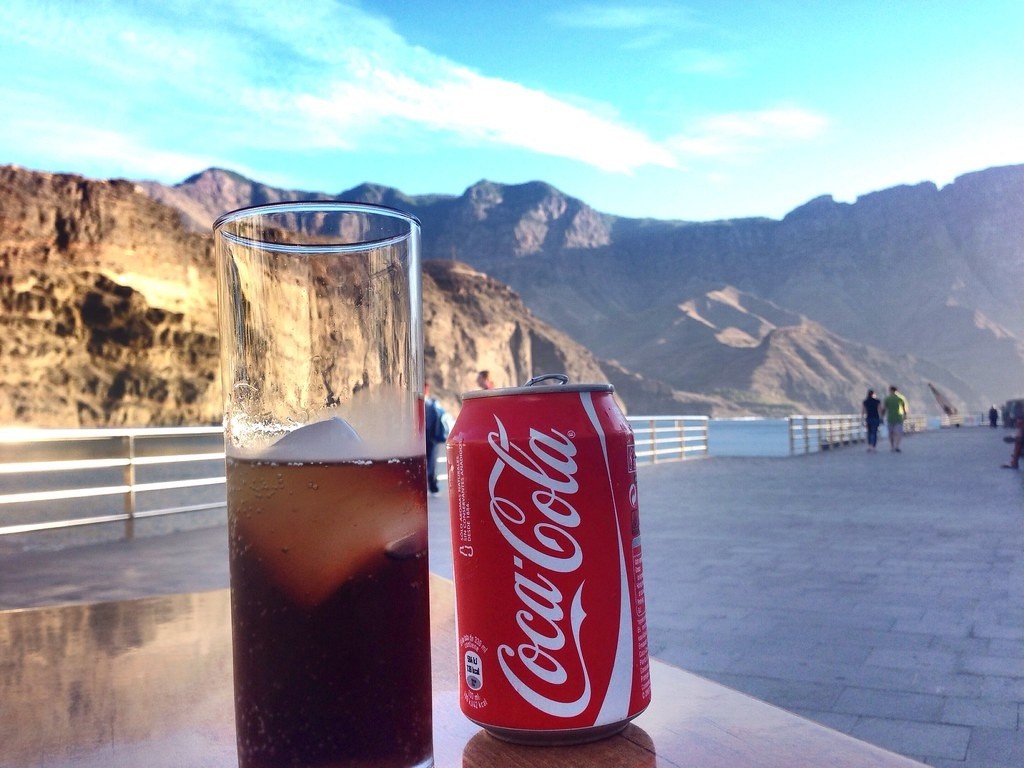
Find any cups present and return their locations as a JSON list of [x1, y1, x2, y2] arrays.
[[212, 200, 434, 768]]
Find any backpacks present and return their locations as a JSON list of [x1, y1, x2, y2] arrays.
[[429, 398, 456, 443]]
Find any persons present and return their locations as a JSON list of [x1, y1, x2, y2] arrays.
[[881, 383, 907, 452], [423, 382, 449, 493], [999, 415, 1024, 470], [988, 404, 998, 428], [861, 388, 882, 453], [476, 370, 496, 389]]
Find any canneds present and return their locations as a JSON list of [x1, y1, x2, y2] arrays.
[[444, 373, 652, 746]]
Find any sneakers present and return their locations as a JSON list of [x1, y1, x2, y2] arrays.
[[432, 491, 443, 499]]
